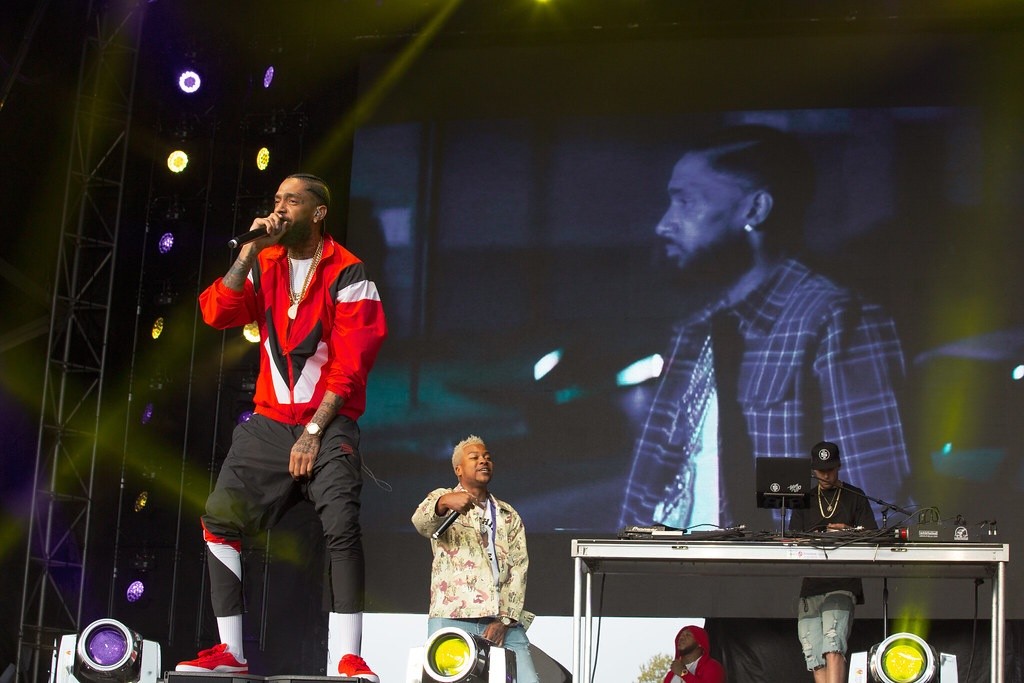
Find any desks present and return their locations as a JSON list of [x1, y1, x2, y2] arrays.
[[569, 537, 1010, 683]]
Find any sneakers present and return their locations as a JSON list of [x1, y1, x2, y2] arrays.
[[338, 654, 380, 683], [175, 643, 248, 674]]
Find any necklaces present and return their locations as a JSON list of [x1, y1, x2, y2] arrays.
[[480, 497, 488, 534], [818, 480, 842, 518], [821, 488, 838, 512], [287, 240, 322, 319]]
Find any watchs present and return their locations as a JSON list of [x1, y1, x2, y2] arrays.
[[680, 669, 689, 677], [498, 616, 512, 626], [306, 423, 323, 437]]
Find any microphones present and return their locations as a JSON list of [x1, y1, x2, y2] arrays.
[[228, 227, 267, 249], [432, 511, 461, 539]]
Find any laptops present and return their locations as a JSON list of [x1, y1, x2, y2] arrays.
[[755, 456, 812, 509]]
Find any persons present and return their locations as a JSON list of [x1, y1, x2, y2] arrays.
[[662, 624, 726, 683], [175, 172, 389, 683], [787, 441, 879, 683], [410, 433, 539, 683], [617, 119, 920, 530]]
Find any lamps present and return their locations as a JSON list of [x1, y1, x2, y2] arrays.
[[49, 618, 162, 683], [848, 631, 959, 683], [419, 626, 518, 683]]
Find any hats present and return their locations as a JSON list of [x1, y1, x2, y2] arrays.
[[808, 441, 841, 470]]
